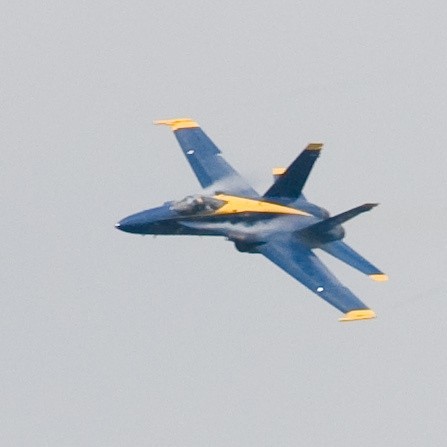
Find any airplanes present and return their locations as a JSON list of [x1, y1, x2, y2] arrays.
[[113, 117, 390, 322]]
